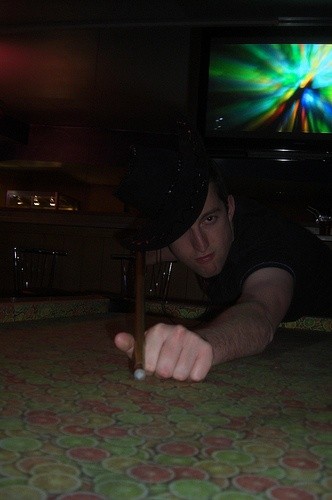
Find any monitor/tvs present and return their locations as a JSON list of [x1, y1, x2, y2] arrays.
[[195, 28, 332, 150]]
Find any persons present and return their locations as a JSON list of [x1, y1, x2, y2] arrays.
[[116, 160, 331, 382]]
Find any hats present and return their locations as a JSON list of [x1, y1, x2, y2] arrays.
[[112, 116, 209, 250]]
